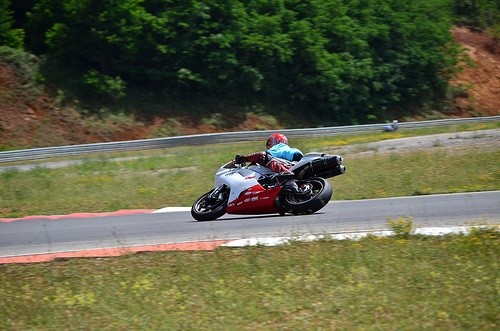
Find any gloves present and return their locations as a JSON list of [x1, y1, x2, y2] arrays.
[[234, 155, 245, 164]]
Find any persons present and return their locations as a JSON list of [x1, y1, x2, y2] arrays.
[[235, 134, 303, 179]]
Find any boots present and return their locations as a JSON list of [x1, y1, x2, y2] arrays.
[[266, 159, 295, 182]]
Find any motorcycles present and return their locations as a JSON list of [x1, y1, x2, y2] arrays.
[[190, 153, 345, 220]]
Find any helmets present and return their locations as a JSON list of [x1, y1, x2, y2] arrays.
[[265, 133, 288, 149]]
